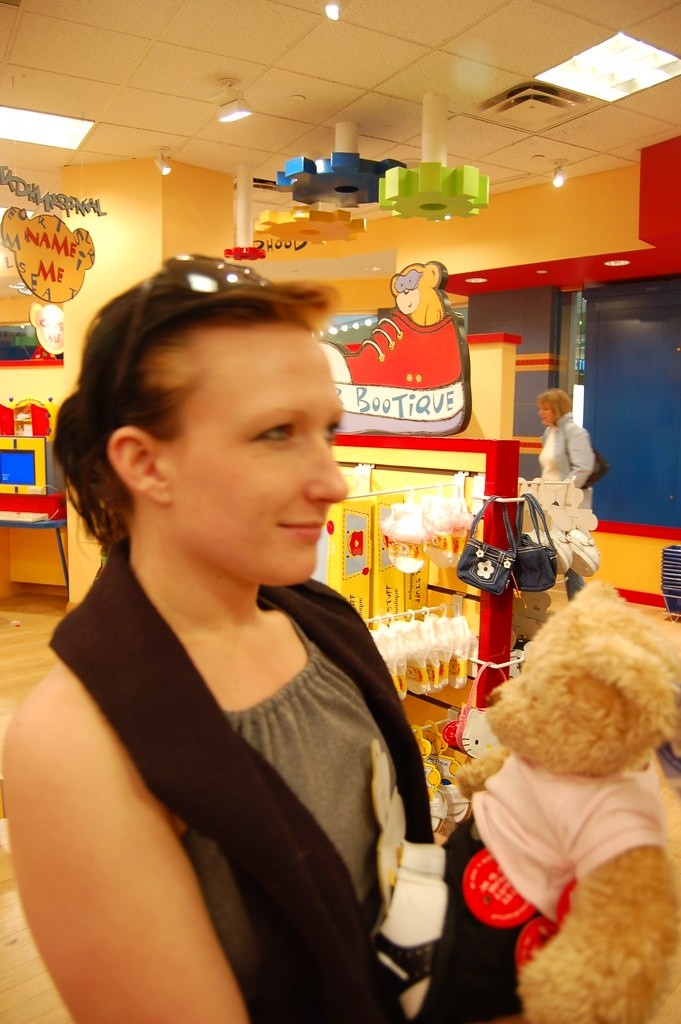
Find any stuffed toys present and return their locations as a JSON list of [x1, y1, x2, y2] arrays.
[[456, 578, 681, 1024]]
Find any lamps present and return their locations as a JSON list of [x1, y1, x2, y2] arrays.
[[551, 158, 569, 188], [325, 0, 350, 22], [208, 79, 252, 122], [154, 145, 171, 176]]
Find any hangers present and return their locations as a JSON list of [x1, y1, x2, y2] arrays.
[[370, 603, 459, 628]]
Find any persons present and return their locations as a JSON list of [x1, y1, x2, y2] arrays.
[[0, 255, 435, 1024], [536, 387, 611, 601]]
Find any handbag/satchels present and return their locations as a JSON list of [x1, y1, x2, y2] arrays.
[[456, 495, 517, 596], [562, 418, 611, 492], [508, 493, 558, 593]]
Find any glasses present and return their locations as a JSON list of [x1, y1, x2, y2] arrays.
[[113, 253, 274, 400]]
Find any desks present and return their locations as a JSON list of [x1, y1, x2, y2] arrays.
[[0, 520, 68, 594]]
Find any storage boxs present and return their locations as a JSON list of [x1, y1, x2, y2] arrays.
[[659, 545, 681, 613], [0, 492, 67, 520], [324, 492, 428, 630]]
